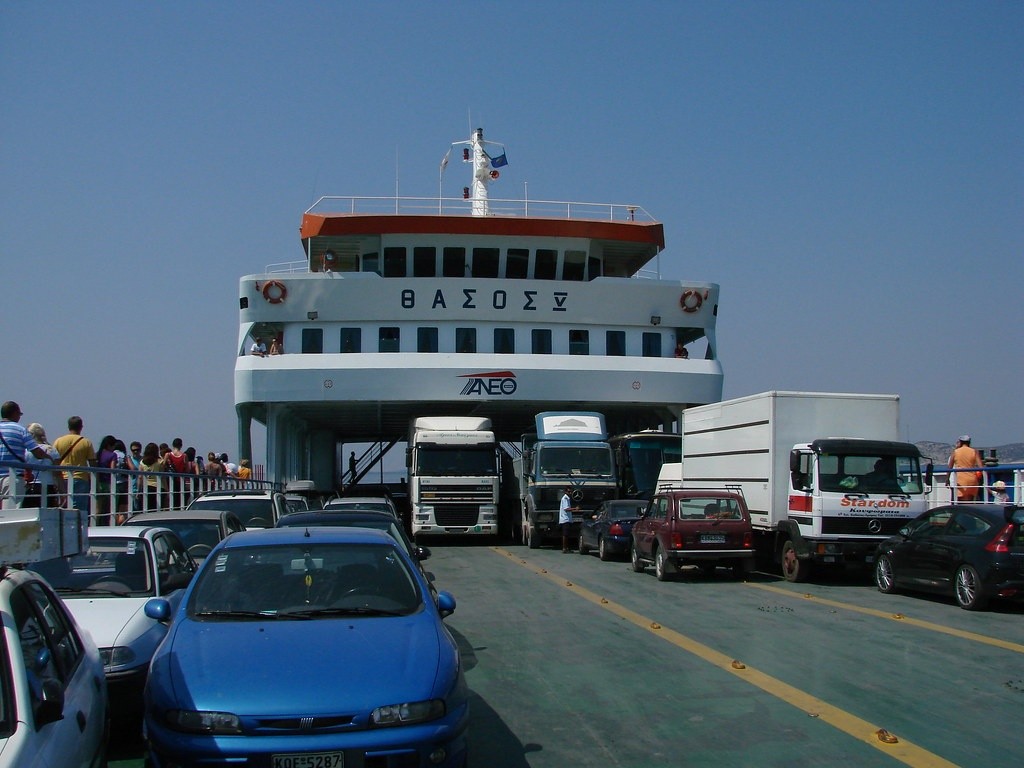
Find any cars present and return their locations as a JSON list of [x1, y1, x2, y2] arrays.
[[139, 527, 469, 768], [577, 500, 652, 561], [119, 510, 252, 571], [271, 494, 402, 536], [875, 505, 1024, 611], [183, 490, 291, 538], [0, 564, 112, 768], [632, 491, 755, 581], [14, 526, 206, 730]]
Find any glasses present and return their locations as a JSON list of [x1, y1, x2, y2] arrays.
[[18, 412, 23, 416]]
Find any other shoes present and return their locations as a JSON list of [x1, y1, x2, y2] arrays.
[[563, 548, 574, 554]]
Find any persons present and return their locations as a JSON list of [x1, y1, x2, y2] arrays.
[[675, 342, 688, 358], [24, 416, 253, 526], [251, 337, 266, 357], [0, 401, 50, 509], [945, 435, 984, 504], [983, 481, 1009, 504], [559, 487, 582, 553], [347, 452, 361, 483], [861, 460, 903, 493], [270, 337, 284, 355]]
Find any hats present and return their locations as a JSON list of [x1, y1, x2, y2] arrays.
[[993, 481, 1005, 490], [959, 435, 970, 441]]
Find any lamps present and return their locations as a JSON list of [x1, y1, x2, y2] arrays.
[[650, 314, 662, 326], [307, 311, 318, 321]]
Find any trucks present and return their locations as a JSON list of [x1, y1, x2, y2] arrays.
[[505, 411, 622, 548], [681, 392, 934, 585], [607, 434, 684, 495], [412, 417, 499, 538]]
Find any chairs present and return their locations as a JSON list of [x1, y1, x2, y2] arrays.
[[703, 504, 721, 517], [246, 569, 284, 606], [958, 515, 977, 533], [345, 564, 383, 598], [114, 551, 146, 588]]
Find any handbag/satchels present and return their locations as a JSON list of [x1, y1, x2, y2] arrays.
[[22, 485, 41, 506], [188, 460, 195, 478], [164, 453, 177, 480]]
